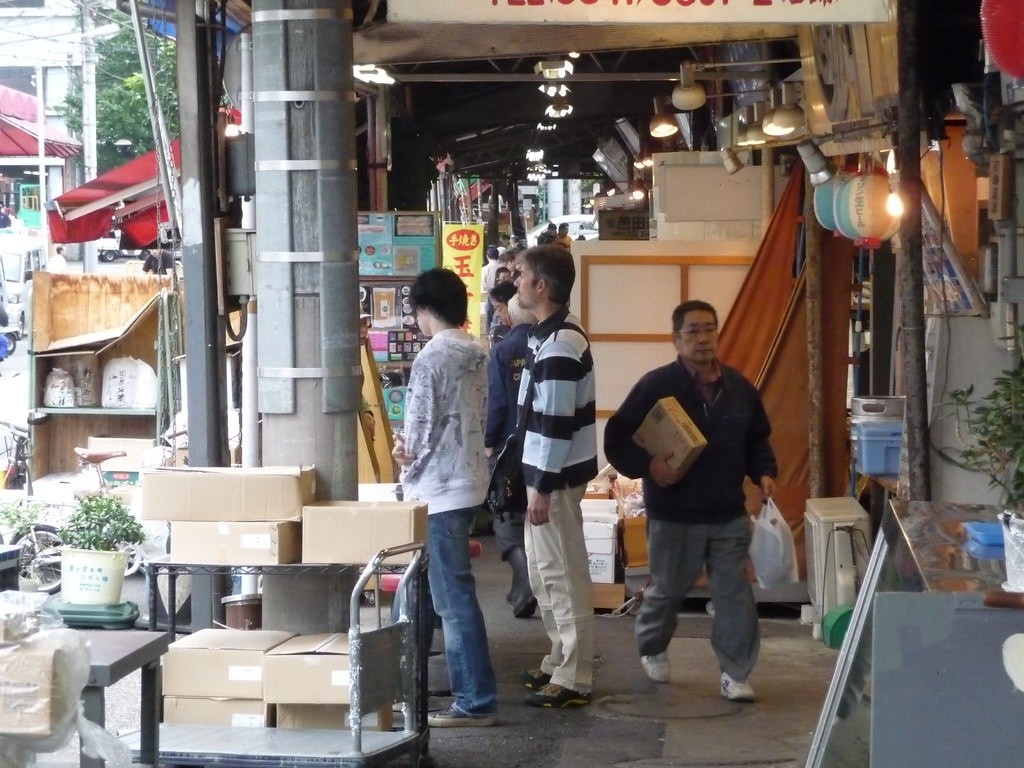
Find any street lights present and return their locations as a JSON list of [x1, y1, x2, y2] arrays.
[[34, 22, 120, 271]]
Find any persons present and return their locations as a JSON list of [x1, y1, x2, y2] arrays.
[[481, 223, 586, 346], [483, 293, 540, 619], [603, 300, 777, 700], [514, 244, 598, 708], [142, 249, 173, 274], [46, 246, 66, 274], [0, 200, 11, 229], [390, 268, 501, 728]]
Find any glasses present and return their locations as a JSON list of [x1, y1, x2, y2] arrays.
[[677, 325, 717, 340]]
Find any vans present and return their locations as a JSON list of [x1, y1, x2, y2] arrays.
[[0, 231, 50, 359]]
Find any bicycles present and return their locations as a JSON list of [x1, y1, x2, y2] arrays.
[[0, 417, 30, 490], [8, 431, 192, 594]]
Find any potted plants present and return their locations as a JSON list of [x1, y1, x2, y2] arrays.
[[61, 492, 143, 605]]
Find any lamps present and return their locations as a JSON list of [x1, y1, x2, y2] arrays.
[[525, 56, 575, 181], [632, 54, 838, 199]]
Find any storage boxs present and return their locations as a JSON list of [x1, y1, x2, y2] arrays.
[[578, 397, 707, 612], [857, 419, 903, 475], [132, 465, 430, 566], [159, 626, 401, 732], [0, 636, 73, 738]]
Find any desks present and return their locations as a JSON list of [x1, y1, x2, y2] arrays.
[[75, 627, 170, 767], [890, 489, 1024, 593]]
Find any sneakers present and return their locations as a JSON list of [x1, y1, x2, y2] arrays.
[[392, 698, 428, 712], [716, 672, 756, 703], [521, 669, 550, 689], [426, 705, 499, 727], [641, 650, 671, 683], [525, 681, 591, 708]]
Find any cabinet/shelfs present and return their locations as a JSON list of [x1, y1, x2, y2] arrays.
[[29, 270, 180, 508]]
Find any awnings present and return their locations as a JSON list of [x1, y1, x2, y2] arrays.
[[0, 84, 83, 157], [44, 107, 242, 249]]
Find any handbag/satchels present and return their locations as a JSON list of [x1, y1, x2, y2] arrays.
[[485, 433, 528, 524], [747, 497, 800, 590]]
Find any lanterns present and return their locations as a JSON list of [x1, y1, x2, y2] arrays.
[[814, 171, 853, 237], [834, 171, 901, 249], [979, 0, 1024, 79]]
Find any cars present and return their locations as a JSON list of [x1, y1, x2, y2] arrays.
[[526, 213, 599, 249], [97, 229, 182, 263]]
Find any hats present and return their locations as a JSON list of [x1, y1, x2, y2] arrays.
[[360, 302, 373, 319], [487, 247, 499, 259]]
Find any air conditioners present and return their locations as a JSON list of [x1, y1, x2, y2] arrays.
[[804, 497, 872, 617]]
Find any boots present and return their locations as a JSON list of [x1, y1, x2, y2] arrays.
[[506, 545, 537, 618]]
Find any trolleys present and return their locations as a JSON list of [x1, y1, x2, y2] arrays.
[[104, 541, 439, 768]]
